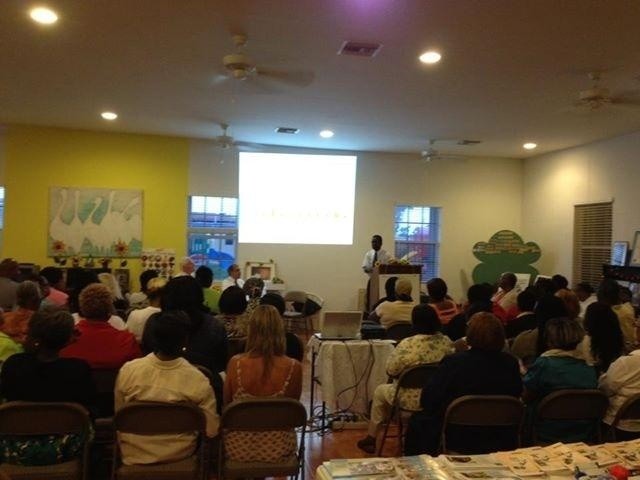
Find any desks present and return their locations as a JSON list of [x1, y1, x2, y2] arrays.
[[315, 440, 640, 480], [311, 339, 401, 436]]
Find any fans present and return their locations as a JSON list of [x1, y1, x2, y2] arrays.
[[178, 34, 316, 86], [405, 134, 470, 168], [559, 71, 640, 115], [203, 120, 271, 156]]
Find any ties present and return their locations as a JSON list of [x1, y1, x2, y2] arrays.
[[234, 280, 239, 287], [373, 252, 378, 262]]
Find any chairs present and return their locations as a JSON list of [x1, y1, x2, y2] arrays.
[[383, 320, 640, 454], [0, 288, 324, 480]]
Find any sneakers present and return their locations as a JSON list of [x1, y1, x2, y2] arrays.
[[358, 439, 375, 453]]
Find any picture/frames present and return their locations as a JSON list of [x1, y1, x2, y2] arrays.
[[612, 240, 630, 266], [629, 229, 640, 267], [246, 261, 278, 282]]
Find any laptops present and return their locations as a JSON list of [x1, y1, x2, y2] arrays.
[[316, 310, 364, 341]]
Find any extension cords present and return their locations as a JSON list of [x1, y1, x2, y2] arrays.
[[334, 418, 371, 430]]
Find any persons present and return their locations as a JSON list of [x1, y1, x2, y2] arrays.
[[362, 235, 395, 312]]
